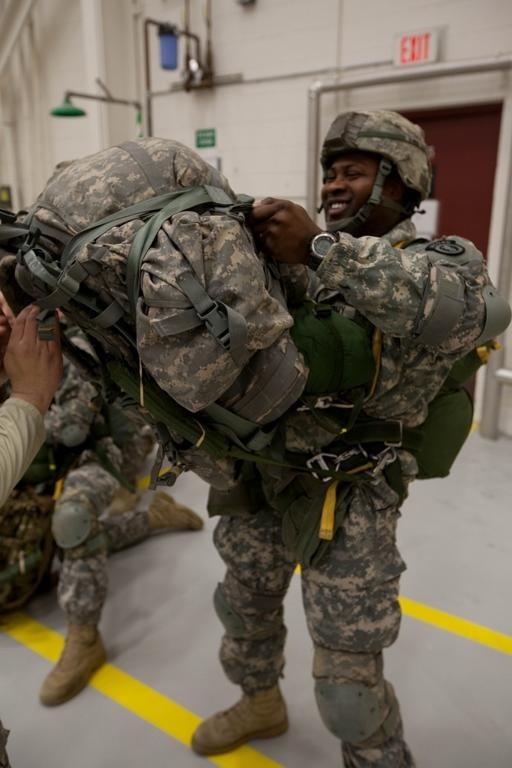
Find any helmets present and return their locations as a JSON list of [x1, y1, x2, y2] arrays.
[[321, 108, 432, 198]]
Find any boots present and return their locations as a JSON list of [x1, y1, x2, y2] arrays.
[[146, 491, 203, 532], [39, 618, 105, 705], [192, 683, 287, 756]]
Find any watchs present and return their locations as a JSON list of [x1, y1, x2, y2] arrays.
[[305, 227, 341, 272]]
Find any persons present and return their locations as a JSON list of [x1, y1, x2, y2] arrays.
[[180, 105, 510, 768], [2, 307, 210, 706], [1, 297, 64, 768]]
